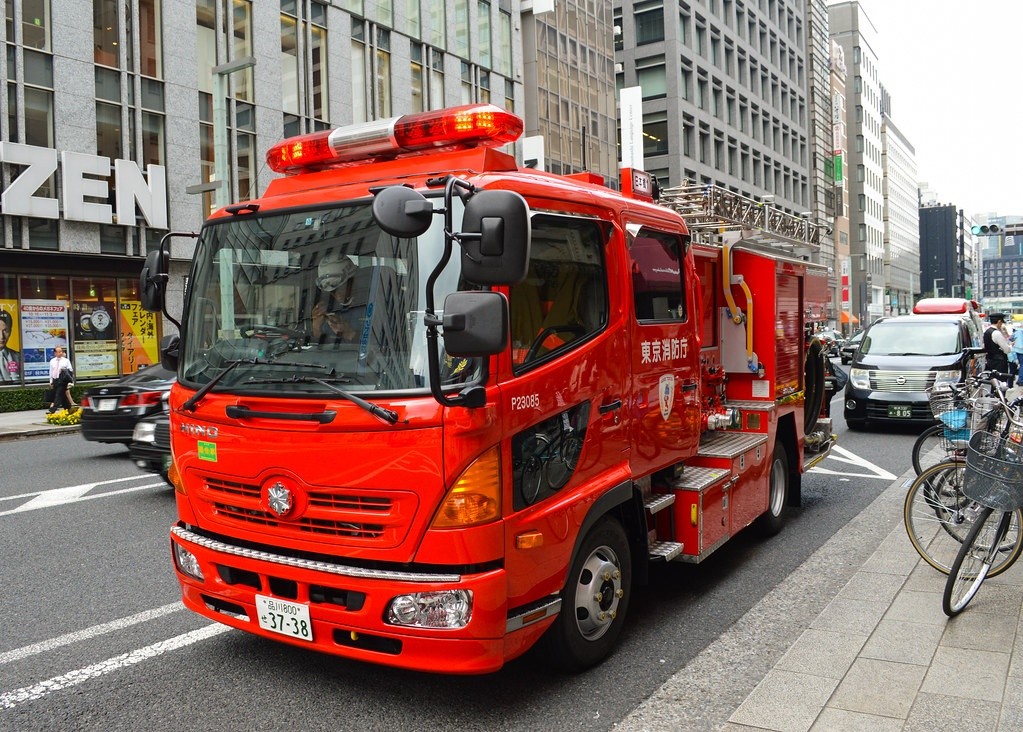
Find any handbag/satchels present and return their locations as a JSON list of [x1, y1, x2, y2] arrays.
[[830, 363, 848, 390], [44, 390, 56, 402]]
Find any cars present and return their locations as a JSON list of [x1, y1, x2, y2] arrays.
[[838, 329, 867, 365], [80, 362, 177, 449], [812, 330, 847, 356], [129, 409, 176, 488]]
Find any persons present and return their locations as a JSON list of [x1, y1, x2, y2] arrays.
[[310, 254, 369, 344], [982, 313, 1011, 400], [1002, 319, 1023, 391], [45, 346, 78, 414], [0, 308, 21, 381]]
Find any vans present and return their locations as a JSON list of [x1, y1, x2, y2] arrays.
[[843, 314, 976, 434], [909, 297, 985, 372]]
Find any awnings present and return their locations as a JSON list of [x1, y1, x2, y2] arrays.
[[841, 311, 858, 323]]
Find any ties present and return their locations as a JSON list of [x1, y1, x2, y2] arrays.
[[54, 358, 59, 379], [0, 352, 11, 381]]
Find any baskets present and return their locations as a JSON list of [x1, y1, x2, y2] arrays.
[[925, 385, 995, 451], [962, 430, 1023, 513]]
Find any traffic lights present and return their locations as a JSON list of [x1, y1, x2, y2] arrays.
[[971, 224, 1001, 236]]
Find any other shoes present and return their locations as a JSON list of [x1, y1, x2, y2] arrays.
[[46, 411, 53, 414]]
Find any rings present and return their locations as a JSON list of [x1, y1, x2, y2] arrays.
[[316, 305, 319, 309]]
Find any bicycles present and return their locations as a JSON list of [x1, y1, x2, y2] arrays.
[[902, 369, 1023, 618]]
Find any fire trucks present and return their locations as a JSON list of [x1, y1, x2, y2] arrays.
[[139, 103, 835, 680]]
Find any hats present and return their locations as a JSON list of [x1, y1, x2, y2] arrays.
[[988, 313, 1006, 323]]
